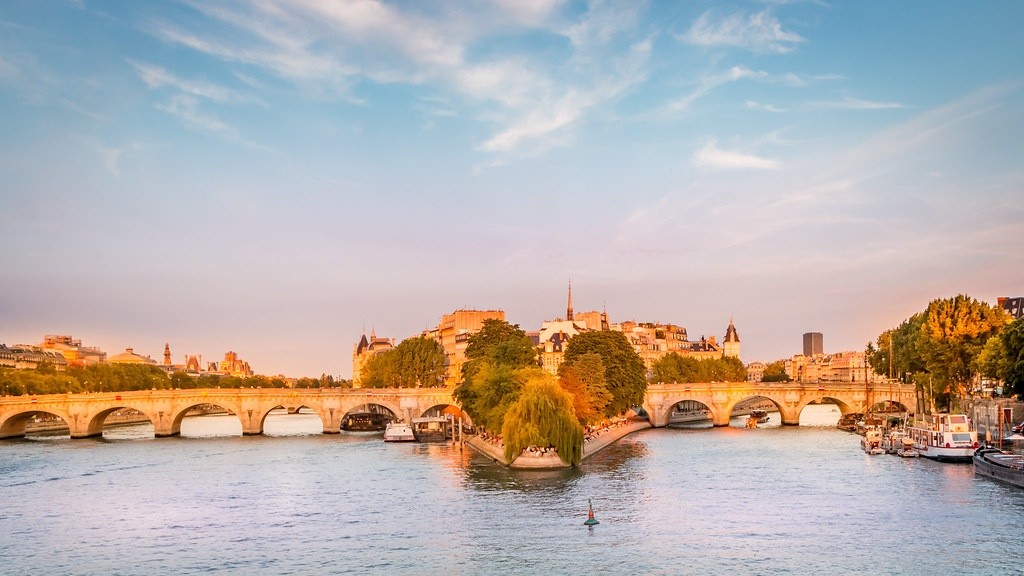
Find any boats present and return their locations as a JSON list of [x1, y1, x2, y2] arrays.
[[340, 410, 447, 443], [750, 409, 770, 423], [836, 333, 1024, 489], [745, 418, 757, 429]]
